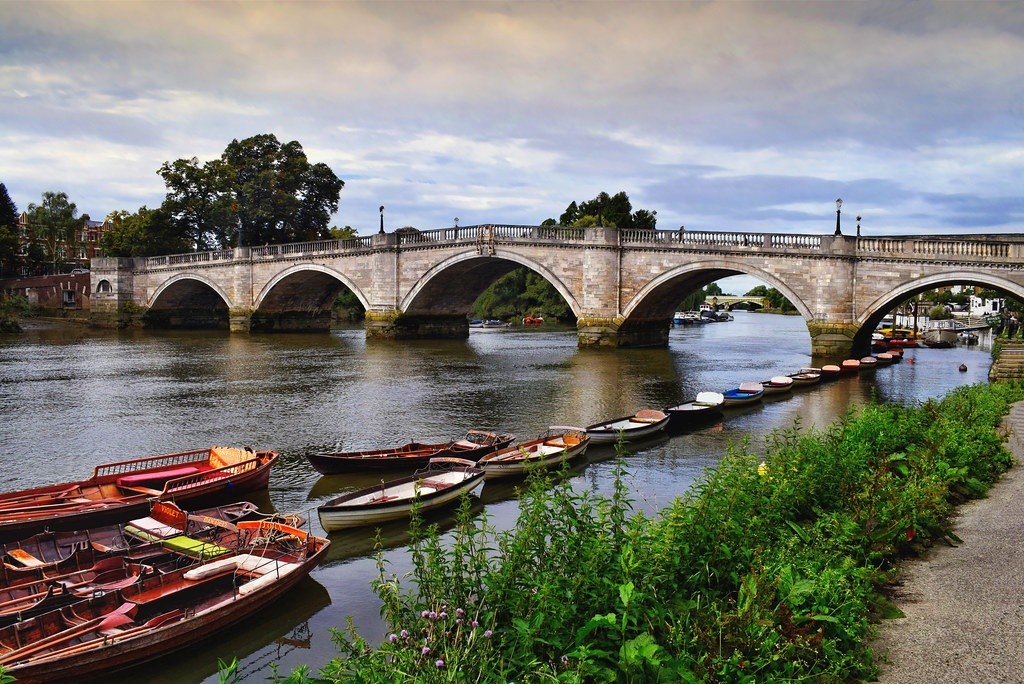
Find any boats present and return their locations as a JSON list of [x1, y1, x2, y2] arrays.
[[0, 498, 331, 684], [469, 319, 504, 328], [816, 364, 841, 380], [52, 573, 332, 684], [317, 493, 484, 567], [788, 368, 823, 385], [587, 409, 671, 446], [0, 444, 278, 547], [664, 391, 726, 423], [585, 430, 671, 463], [306, 430, 516, 477], [673, 304, 734, 324], [316, 456, 487, 536], [480, 456, 590, 506], [760, 375, 794, 394], [721, 381, 766, 408], [841, 328, 957, 374], [479, 424, 591, 482]]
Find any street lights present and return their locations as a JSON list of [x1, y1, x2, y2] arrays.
[[380, 204, 386, 234], [236, 218, 243, 247], [835, 198, 844, 235], [596, 195, 604, 227], [454, 217, 460, 240], [855, 215, 862, 236]]
